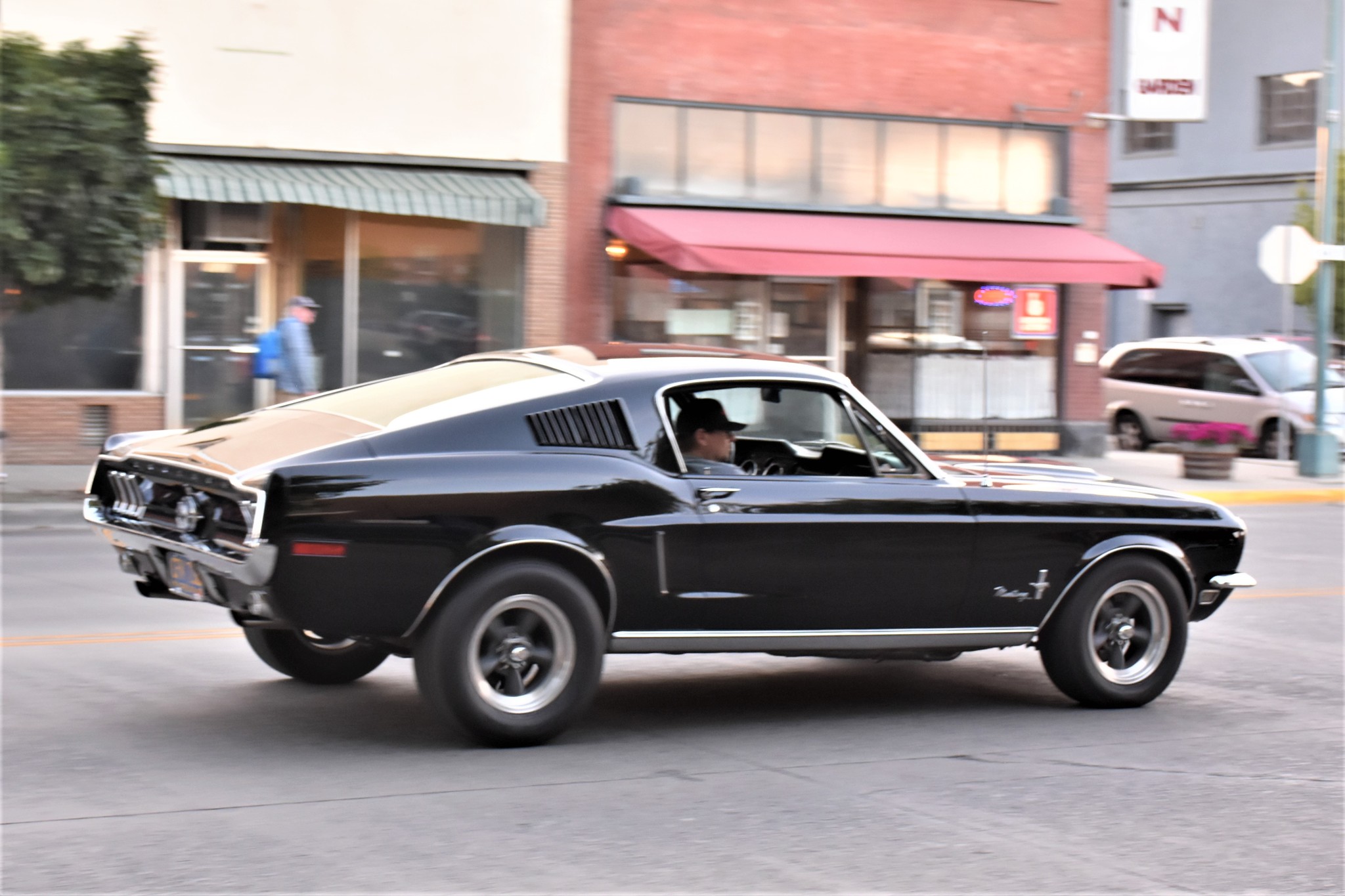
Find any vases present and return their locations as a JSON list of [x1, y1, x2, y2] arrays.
[[1182, 441, 1238, 480]]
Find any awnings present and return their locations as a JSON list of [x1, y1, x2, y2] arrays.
[[146, 155, 548, 230], [605, 206, 1163, 289]]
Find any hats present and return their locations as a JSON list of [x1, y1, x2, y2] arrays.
[[677, 398, 747, 431], [288, 295, 322, 309]]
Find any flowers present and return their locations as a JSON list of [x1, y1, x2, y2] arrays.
[[1172, 423, 1258, 443]]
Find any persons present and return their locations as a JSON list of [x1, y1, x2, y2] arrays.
[[675, 397, 749, 476], [280, 296, 323, 402]]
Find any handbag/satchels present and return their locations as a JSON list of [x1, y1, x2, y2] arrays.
[[253, 331, 282, 377]]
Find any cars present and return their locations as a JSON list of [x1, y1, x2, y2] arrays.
[[74, 345, 1258, 751], [1097, 333, 1345, 463]]
